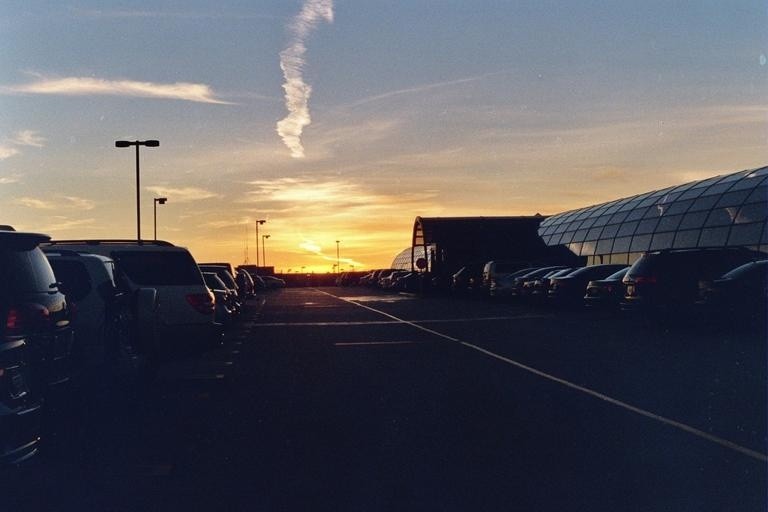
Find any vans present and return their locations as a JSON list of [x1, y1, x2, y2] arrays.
[[37, 238, 215, 388]]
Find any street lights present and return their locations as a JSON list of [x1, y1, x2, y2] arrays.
[[154, 197, 167, 241], [256, 220, 265, 267], [336, 240, 340, 276], [115, 140, 160, 240], [262, 235, 270, 267]]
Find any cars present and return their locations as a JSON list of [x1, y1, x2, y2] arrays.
[[693, 258, 768, 331], [193, 259, 287, 345], [333, 260, 632, 352]]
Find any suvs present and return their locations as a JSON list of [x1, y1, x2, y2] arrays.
[[617, 247, 768, 328], [34, 246, 161, 421], [0, 225, 77, 510]]
